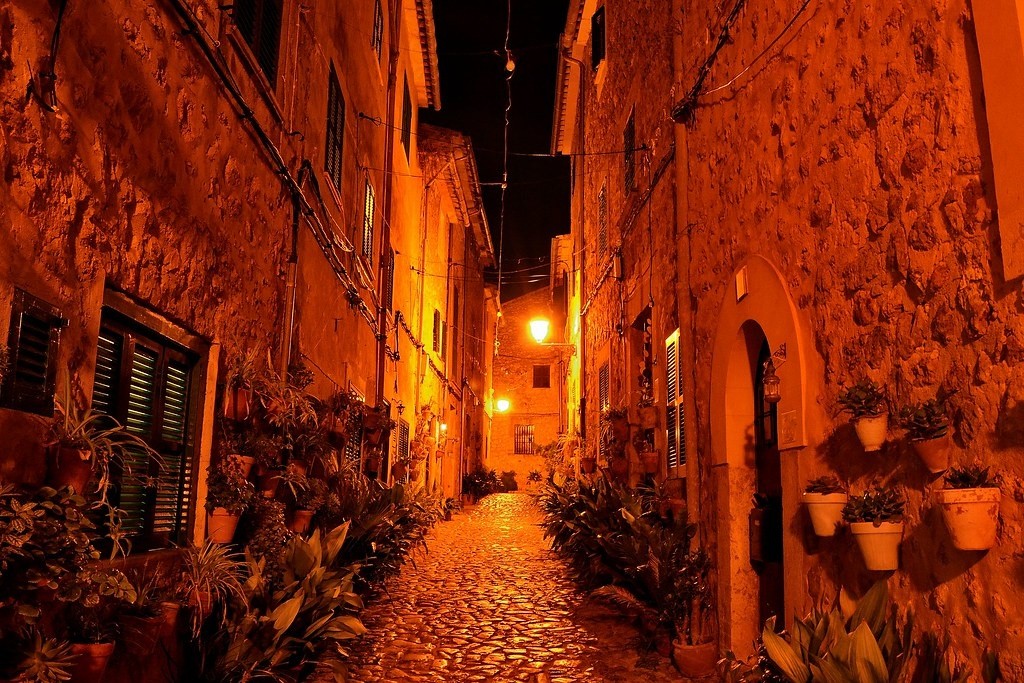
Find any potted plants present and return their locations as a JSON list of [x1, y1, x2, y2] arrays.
[[841, 478, 904, 570], [0, 331, 718, 683], [933, 463, 1004, 551], [803, 474, 848, 536], [836, 373, 890, 451], [899, 396, 955, 475]]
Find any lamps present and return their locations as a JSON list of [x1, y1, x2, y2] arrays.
[[763, 342, 787, 403]]
